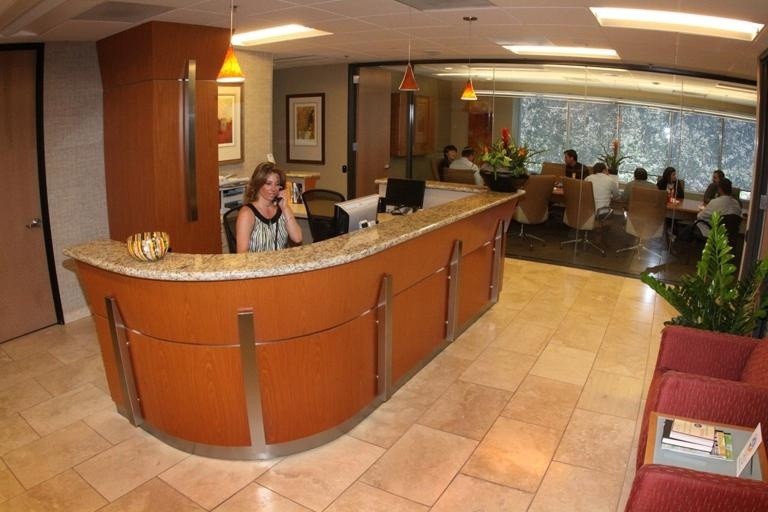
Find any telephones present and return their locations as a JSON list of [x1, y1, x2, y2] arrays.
[[274, 184, 285, 202]]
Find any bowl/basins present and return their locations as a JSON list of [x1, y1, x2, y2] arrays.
[[128, 231, 169, 261]]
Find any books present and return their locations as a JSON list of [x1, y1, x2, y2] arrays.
[[658, 417, 734, 462]]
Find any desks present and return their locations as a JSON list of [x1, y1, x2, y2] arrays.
[[551, 186, 749, 235], [643, 408, 767, 481]]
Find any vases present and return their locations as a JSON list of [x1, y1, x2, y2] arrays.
[[607, 168, 619, 176], [480, 174, 518, 192]]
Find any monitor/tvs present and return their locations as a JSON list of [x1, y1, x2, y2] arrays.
[[739, 190, 751, 201], [333, 193, 380, 236], [385, 178, 426, 215]]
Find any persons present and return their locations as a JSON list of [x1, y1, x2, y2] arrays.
[[620, 167, 658, 211], [448, 146, 485, 186], [438, 144, 457, 182], [233, 161, 304, 255], [562, 148, 589, 180], [584, 161, 620, 218], [674, 177, 740, 242], [702, 169, 725, 203], [656, 166, 685, 199]]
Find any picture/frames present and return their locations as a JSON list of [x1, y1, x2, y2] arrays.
[[284, 91, 326, 165], [218, 81, 246, 167]]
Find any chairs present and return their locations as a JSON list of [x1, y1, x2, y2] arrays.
[[657, 175, 685, 196], [636, 320, 768, 466], [540, 160, 569, 176], [732, 186, 741, 198], [624, 460, 767, 511], [223, 206, 245, 252], [615, 184, 669, 263], [698, 214, 744, 264], [430, 157, 442, 180], [442, 167, 476, 185], [559, 173, 614, 257], [301, 189, 345, 242], [506, 173, 558, 250]]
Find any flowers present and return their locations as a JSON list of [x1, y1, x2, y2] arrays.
[[596, 138, 631, 169], [477, 127, 549, 181]]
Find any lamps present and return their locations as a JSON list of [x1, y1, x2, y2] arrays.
[[460, 16, 479, 101], [396, 6, 423, 93], [213, 0, 248, 85]]
